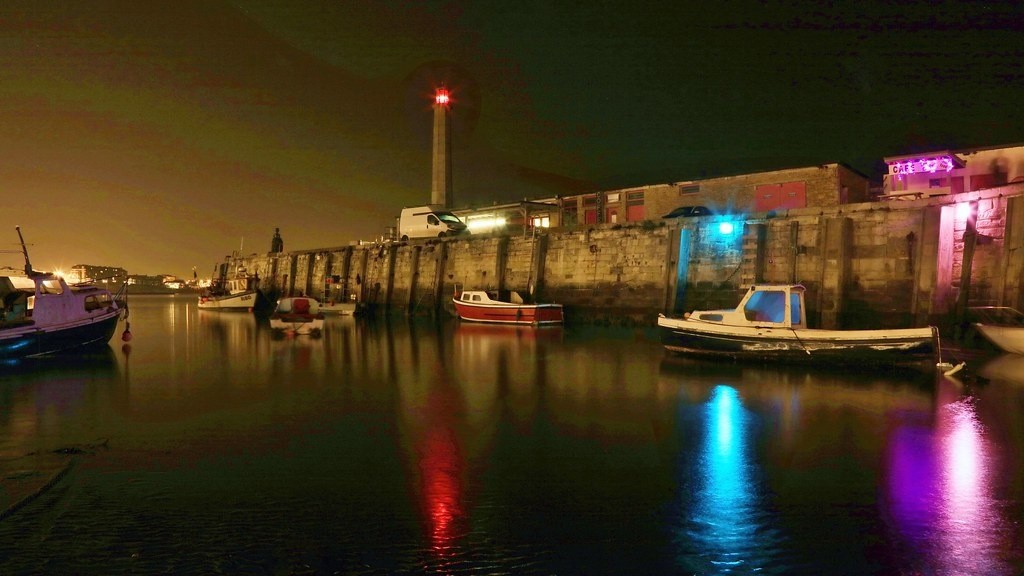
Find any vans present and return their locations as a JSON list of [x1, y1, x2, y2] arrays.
[[399, 203, 471, 242]]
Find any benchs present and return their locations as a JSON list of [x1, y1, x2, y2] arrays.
[[876, 192, 924, 201]]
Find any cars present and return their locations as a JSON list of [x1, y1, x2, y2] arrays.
[[662, 205, 712, 219]]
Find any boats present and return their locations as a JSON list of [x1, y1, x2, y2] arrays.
[[975, 351, 1024, 392], [0, 225, 130, 365], [657, 280, 940, 373], [451, 275, 565, 326], [196, 286, 358, 339], [965, 305, 1024, 357]]
